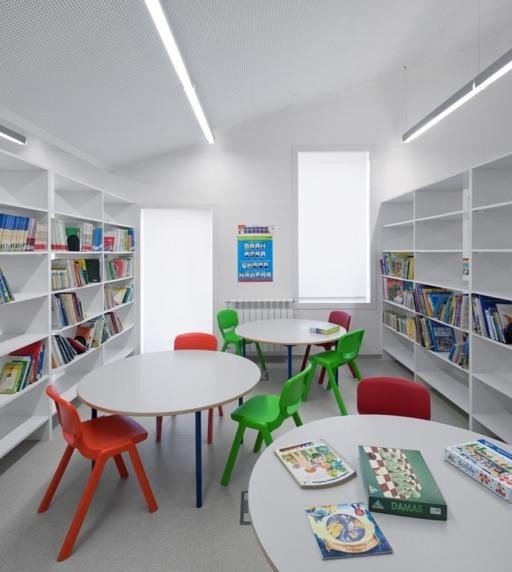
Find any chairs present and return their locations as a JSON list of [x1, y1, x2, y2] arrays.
[[303, 328, 364, 416], [301, 310, 355, 384], [220, 363, 313, 486], [217, 309, 267, 370], [357, 375, 431, 421], [37, 385, 158, 562], [155, 332, 223, 444]]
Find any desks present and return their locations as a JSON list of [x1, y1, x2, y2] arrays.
[[248, 413, 512, 572], [76, 349, 261, 508], [235, 318, 347, 379]]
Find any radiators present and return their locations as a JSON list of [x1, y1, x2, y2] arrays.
[[225, 299, 295, 352]]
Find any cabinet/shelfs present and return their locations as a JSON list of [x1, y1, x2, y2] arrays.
[[103, 190, 138, 365], [50, 172, 104, 421], [1, 149, 50, 450], [380, 152, 512, 444]]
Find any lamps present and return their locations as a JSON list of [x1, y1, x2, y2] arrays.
[[143, 0, 216, 145], [1, 126, 27, 146], [401, 1, 512, 145]]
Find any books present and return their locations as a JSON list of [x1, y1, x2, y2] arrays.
[[0, 214, 135, 393], [273, 437, 357, 489], [304, 502, 393, 561], [381, 252, 512, 369]]
